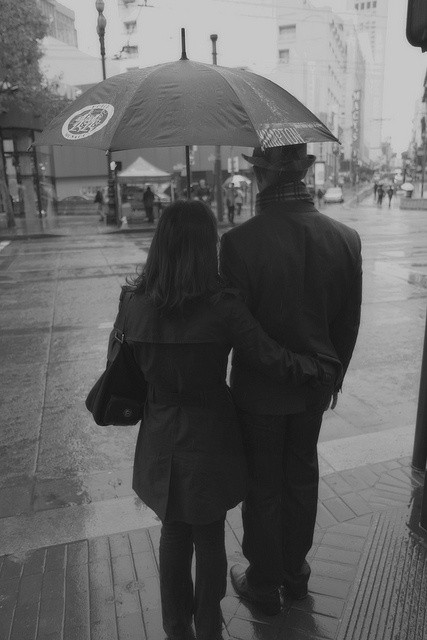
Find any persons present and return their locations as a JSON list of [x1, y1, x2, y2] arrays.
[[142, 186, 155, 222], [218, 143, 363, 615], [226, 183, 237, 223], [376, 187, 383, 208], [107, 202, 339, 640], [386, 185, 393, 208], [317, 189, 321, 207], [373, 182, 376, 199], [405, 192, 412, 198], [236, 190, 243, 216]]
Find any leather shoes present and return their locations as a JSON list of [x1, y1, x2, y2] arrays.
[[230, 563, 282, 614]]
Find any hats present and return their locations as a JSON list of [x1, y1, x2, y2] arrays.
[[241, 142, 317, 173]]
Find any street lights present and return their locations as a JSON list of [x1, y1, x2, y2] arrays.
[[95, 2, 119, 227]]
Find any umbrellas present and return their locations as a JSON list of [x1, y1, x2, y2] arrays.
[[223, 175, 245, 197], [400, 183, 414, 191], [235, 177, 251, 189], [26, 28, 341, 200]]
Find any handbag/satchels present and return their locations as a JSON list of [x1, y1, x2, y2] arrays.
[[85, 286, 147, 427]]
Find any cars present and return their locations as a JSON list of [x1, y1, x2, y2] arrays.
[[324, 187, 343, 203]]
